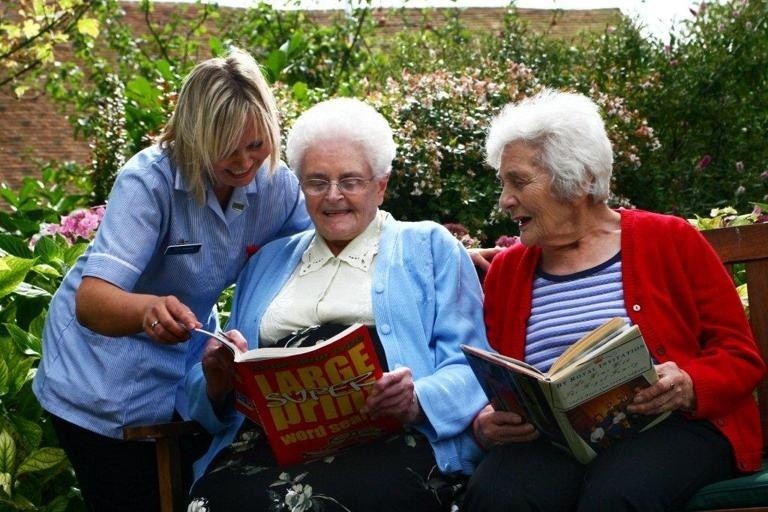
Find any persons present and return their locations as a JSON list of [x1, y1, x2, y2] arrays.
[[31, 51, 314, 512], [186, 98, 500, 512], [457, 89, 768, 511]]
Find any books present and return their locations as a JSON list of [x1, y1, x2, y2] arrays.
[[459, 315, 672, 465], [193, 322, 406, 468]]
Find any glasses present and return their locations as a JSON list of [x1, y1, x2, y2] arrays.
[[299, 176, 377, 196]]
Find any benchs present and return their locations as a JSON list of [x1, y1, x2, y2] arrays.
[[127, 225, 767, 512]]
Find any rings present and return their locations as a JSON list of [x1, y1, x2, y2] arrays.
[[151, 321, 159, 328]]
